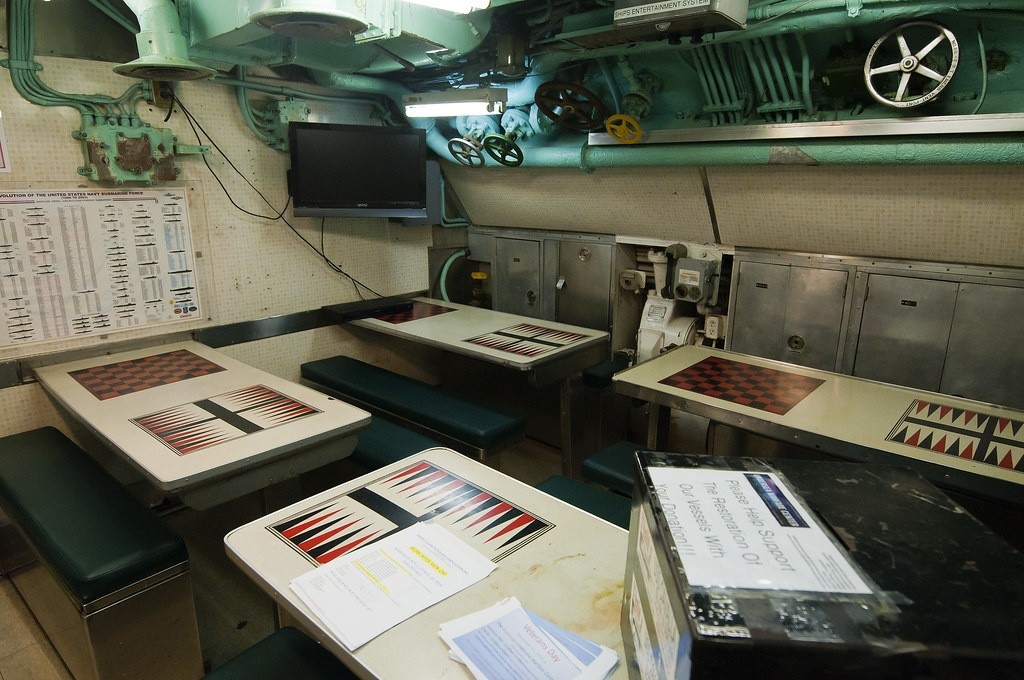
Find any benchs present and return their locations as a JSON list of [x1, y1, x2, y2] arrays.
[[348, 413, 445, 470], [195, 625, 364, 680], [533, 475, 632, 531], [0, 425, 206, 680], [581, 437, 655, 498], [300, 353, 527, 461]]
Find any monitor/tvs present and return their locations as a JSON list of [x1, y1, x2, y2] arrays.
[[288, 120, 428, 209]]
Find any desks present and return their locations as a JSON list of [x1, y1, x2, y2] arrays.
[[33, 339, 372, 634], [612, 341, 1024, 506], [223, 446, 631, 680], [347, 295, 611, 479]]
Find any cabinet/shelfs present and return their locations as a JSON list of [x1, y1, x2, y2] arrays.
[[722, 251, 857, 371], [841, 264, 1024, 412], [488, 234, 542, 323], [556, 240, 614, 343]]
